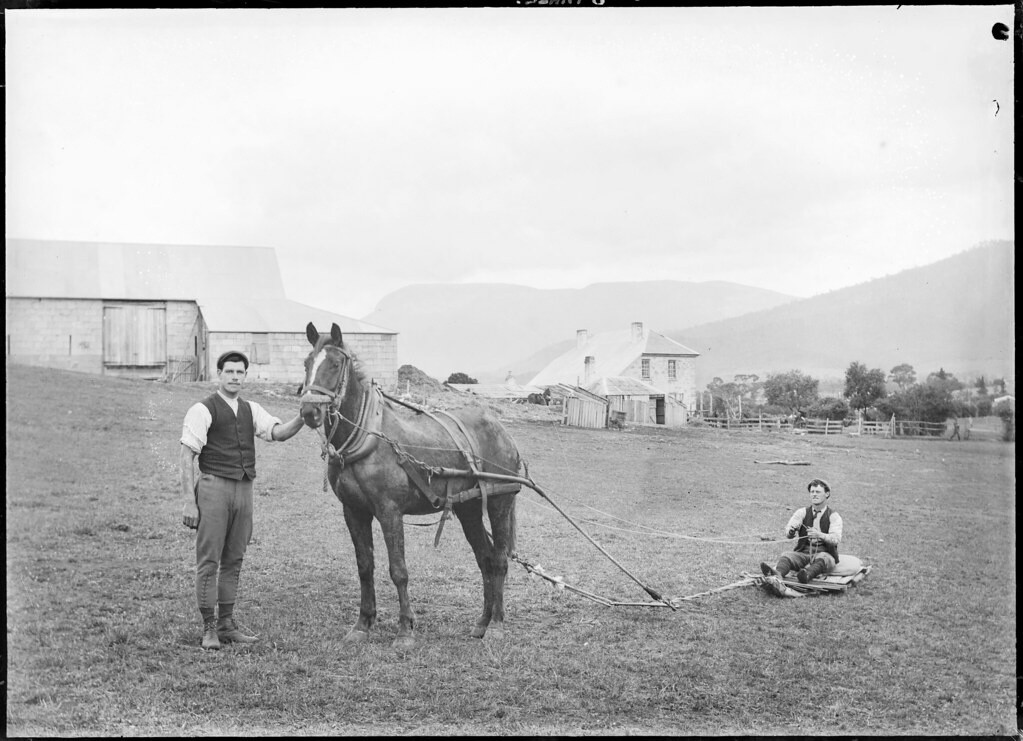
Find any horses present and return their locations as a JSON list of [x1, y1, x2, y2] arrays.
[[300, 322, 525, 648]]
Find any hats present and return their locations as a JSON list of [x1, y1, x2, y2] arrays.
[[217, 351, 249, 369], [807, 478, 830, 499]]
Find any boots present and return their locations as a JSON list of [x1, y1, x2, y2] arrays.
[[202, 614, 221, 650], [797, 558, 826, 583], [217, 615, 260, 643], [761, 557, 792, 580]]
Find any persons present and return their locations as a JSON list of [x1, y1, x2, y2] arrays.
[[179, 351, 305, 649], [543, 387, 551, 405], [760, 479, 843, 584], [964, 414, 976, 441], [948, 414, 961, 442], [780, 411, 805, 428]]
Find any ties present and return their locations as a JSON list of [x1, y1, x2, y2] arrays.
[[812, 510, 821, 519]]
